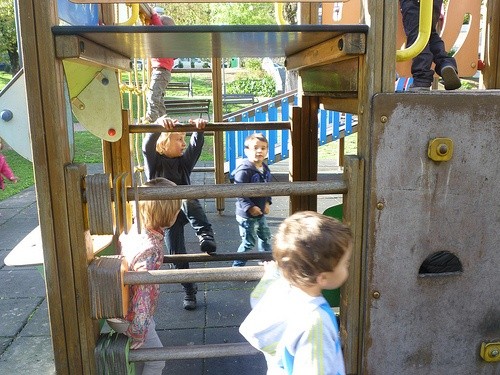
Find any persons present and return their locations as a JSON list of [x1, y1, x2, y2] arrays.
[[122, 179, 184, 375], [136, 7, 176, 124], [0, 137, 18, 194], [231, 133, 275, 268], [238, 211, 353, 375], [141, 112, 216, 310], [403, 0, 459, 91]]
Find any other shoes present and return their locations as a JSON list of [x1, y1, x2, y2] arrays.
[[439, 65, 462, 90], [258, 258, 275, 266], [409, 83, 430, 94]]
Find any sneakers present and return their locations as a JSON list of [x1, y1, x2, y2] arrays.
[[182, 285, 198, 310], [198, 231, 217, 256]]
